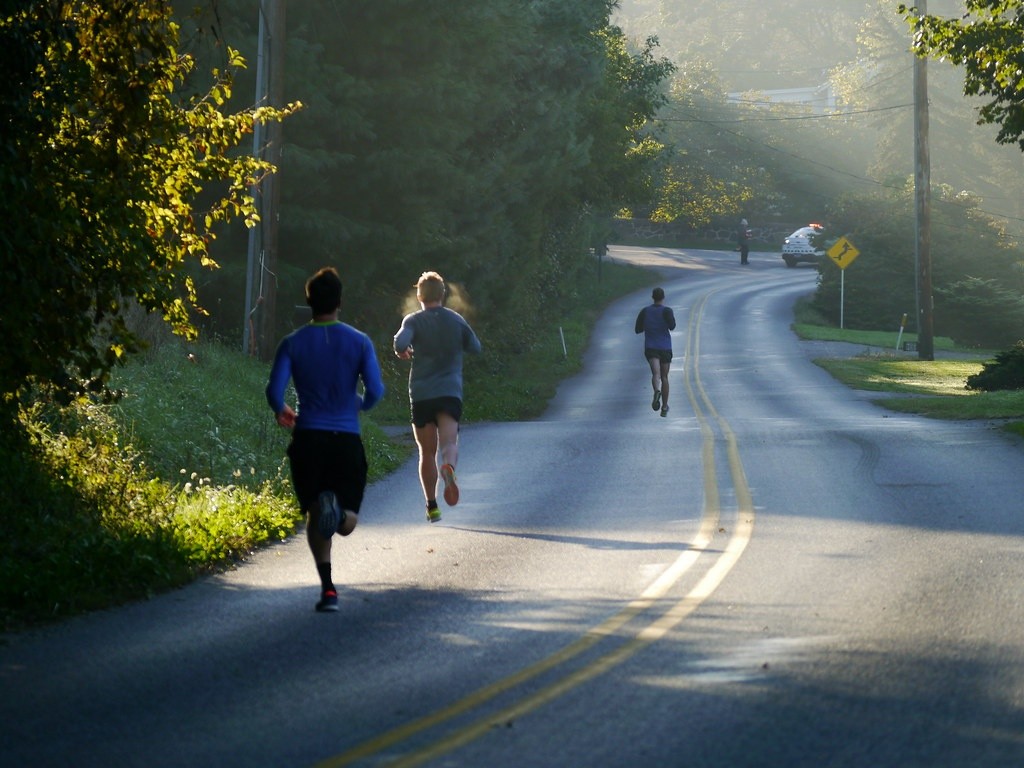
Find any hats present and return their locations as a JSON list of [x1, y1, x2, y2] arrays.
[[739, 219, 749, 227]]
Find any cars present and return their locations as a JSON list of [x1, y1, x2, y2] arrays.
[[782, 223, 840, 268]]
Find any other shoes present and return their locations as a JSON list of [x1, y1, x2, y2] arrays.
[[659, 405, 670, 418], [651, 389, 661, 411]]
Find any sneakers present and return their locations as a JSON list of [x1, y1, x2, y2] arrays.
[[440, 464, 460, 508], [316, 588, 340, 612], [316, 488, 346, 539], [427, 506, 443, 524]]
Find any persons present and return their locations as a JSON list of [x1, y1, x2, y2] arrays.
[[737, 218, 752, 266], [393, 271, 481, 523], [264, 267, 384, 612], [635, 287, 676, 417]]
